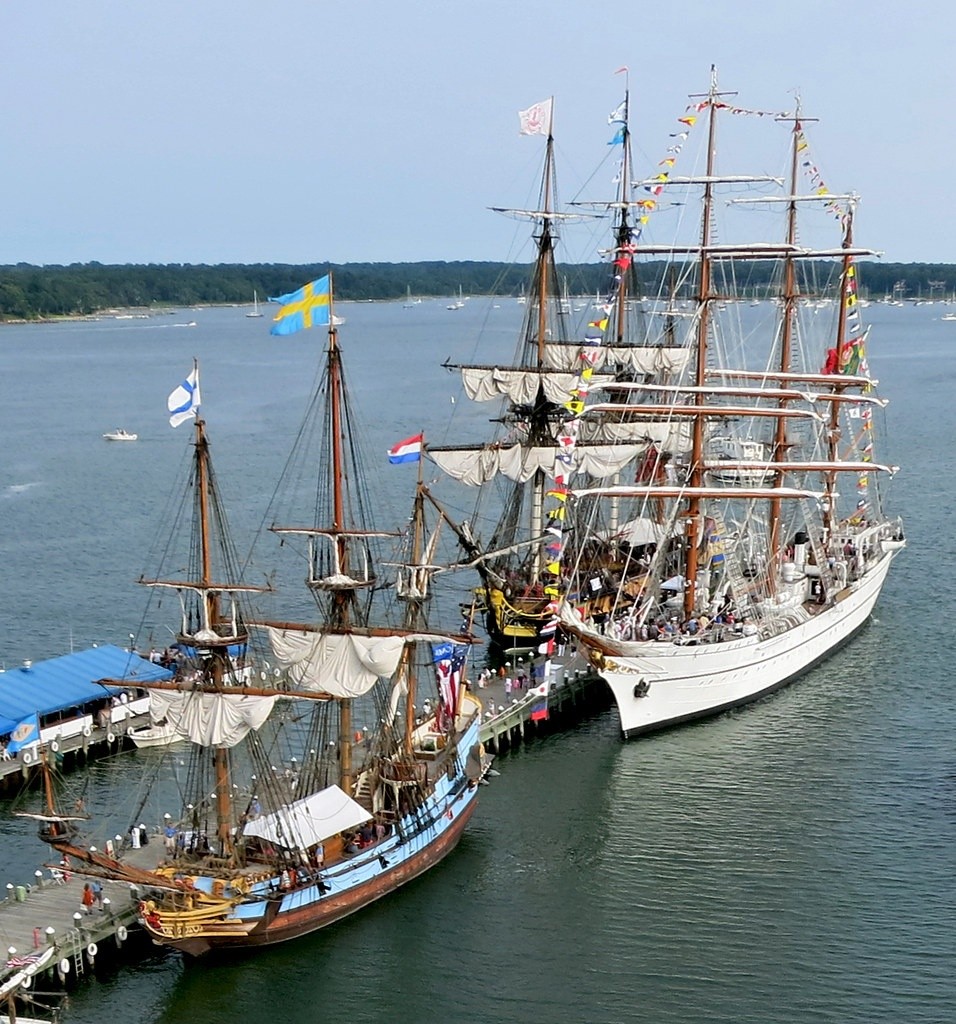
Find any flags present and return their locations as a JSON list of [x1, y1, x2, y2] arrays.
[[519, 98, 552, 136], [167, 370, 202, 428], [270, 275, 332, 336], [793, 123, 845, 219], [387, 433, 422, 465], [608, 101, 627, 124], [541, 369, 593, 554], [637, 101, 707, 210], [580, 216, 649, 363], [2, 713, 39, 753], [531, 561, 562, 719], [433, 644, 468, 729], [715, 102, 791, 118], [842, 255, 874, 521]]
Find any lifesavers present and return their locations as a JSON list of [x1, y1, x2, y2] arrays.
[[263, 662, 269, 670], [246, 678, 252, 687], [61, 958, 70, 973], [273, 668, 280, 677], [51, 741, 58, 751], [127, 726, 134, 735], [88, 943, 97, 955], [83, 728, 91, 737], [107, 733, 115, 742], [19, 969, 31, 988], [118, 926, 127, 940], [23, 753, 32, 763], [261, 671, 267, 680]]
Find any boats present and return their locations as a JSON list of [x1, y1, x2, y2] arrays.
[[102, 432, 138, 441]]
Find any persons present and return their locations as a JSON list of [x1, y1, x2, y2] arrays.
[[314, 819, 385, 868], [618, 614, 730, 639], [815, 582, 821, 603], [163, 823, 178, 855], [77, 878, 106, 914], [478, 634, 577, 714]]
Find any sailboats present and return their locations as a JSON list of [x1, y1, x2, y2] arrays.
[[37, 266, 503, 958], [426, 58, 907, 743]]
